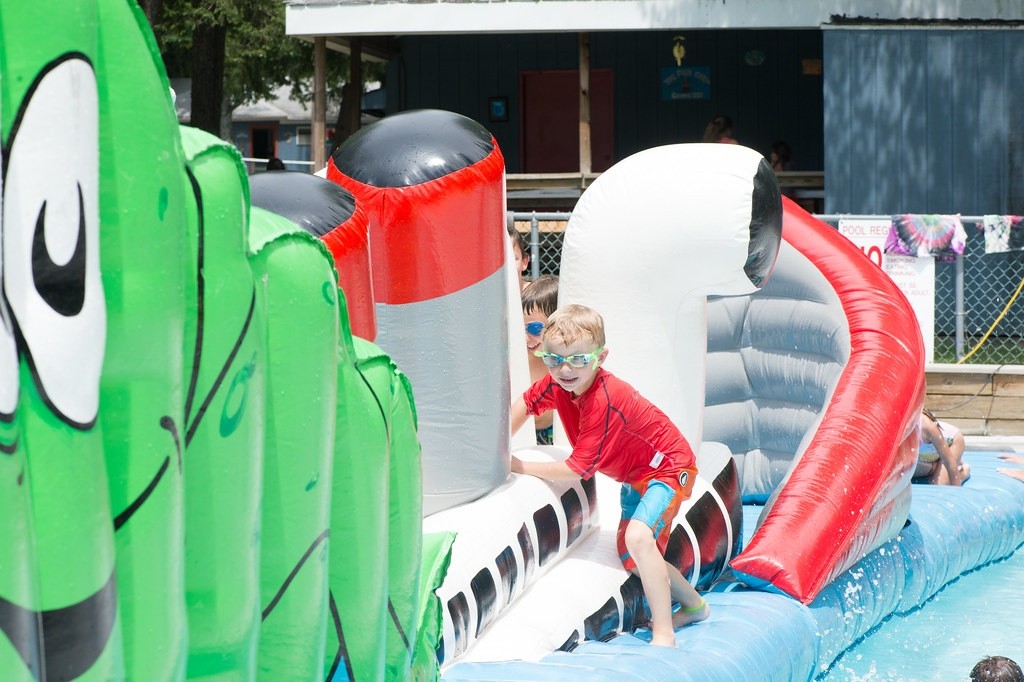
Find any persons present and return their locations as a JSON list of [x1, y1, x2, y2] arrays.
[[919, 409, 970, 486], [510, 304, 710, 649], [509, 230, 531, 292], [996, 454, 1024, 483], [770, 141, 797, 199], [702, 116, 739, 144], [523, 274, 558, 445]]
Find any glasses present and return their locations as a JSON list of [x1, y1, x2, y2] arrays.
[[543, 346, 604, 368], [524, 322, 545, 336]]
